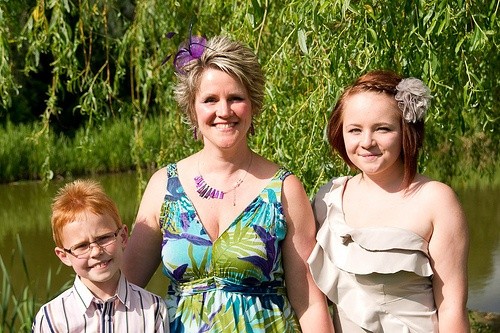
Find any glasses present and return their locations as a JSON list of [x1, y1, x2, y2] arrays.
[[63, 228, 121, 258]]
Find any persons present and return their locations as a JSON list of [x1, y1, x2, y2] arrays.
[[283, 69, 472, 333], [32, 179, 170, 333], [119, 34, 337, 333]]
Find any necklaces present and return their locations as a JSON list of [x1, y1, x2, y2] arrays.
[[194, 149, 253, 206]]
